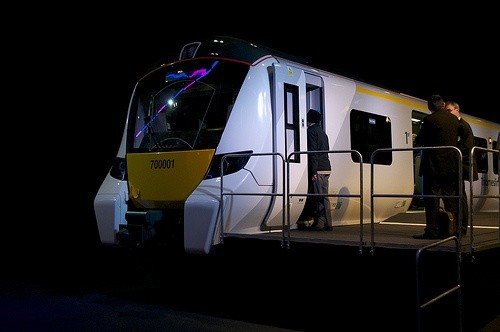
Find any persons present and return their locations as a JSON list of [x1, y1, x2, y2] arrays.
[[442, 102, 480, 236], [412, 94, 462, 239], [306, 108, 333, 231]]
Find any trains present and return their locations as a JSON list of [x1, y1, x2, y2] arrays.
[[93, 35, 500, 255]]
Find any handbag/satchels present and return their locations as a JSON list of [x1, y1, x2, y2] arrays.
[[439, 207, 453, 238]]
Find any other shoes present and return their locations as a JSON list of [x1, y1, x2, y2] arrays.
[[297, 222, 311, 230], [462, 229, 467, 234], [413, 232, 438, 240], [323, 226, 333, 231]]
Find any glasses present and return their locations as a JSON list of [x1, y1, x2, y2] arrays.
[[447, 109, 456, 113]]
